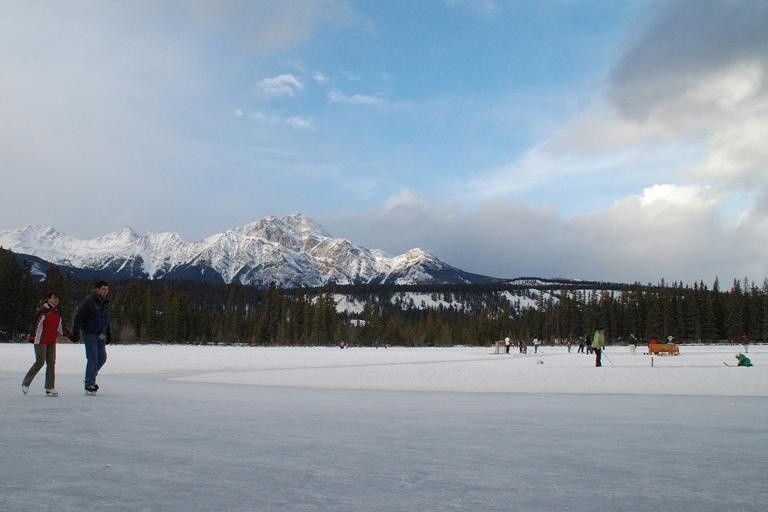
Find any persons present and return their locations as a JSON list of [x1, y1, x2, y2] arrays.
[[736, 352, 753, 367], [590, 325, 607, 367], [504, 333, 593, 354], [628, 332, 638, 352], [665, 329, 676, 354], [70, 279, 112, 393], [647, 333, 659, 355], [22, 292, 77, 395]]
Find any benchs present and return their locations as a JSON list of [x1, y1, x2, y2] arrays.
[[648, 344, 680, 357]]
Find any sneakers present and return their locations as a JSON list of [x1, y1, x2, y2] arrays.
[[23, 384, 29, 393], [85, 384, 98, 392], [46, 389, 58, 394]]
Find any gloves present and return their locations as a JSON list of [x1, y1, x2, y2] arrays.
[[29, 336, 35, 343], [68, 330, 80, 344], [105, 335, 111, 345]]
